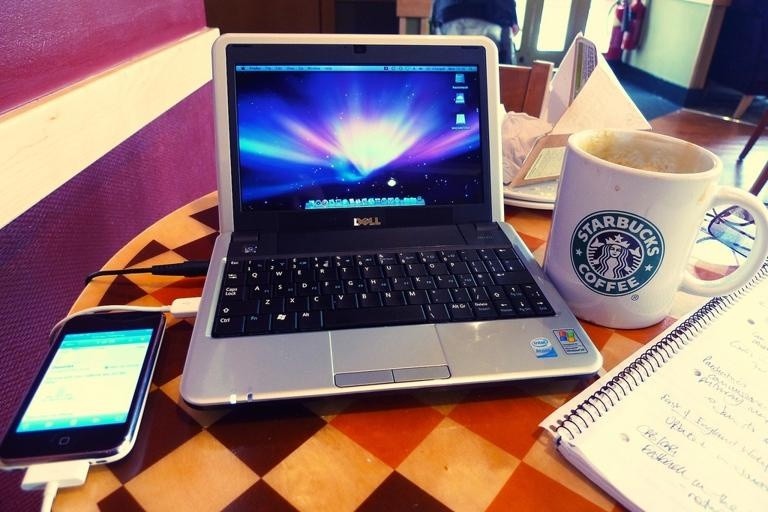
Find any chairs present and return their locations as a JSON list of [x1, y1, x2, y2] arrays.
[[499, 60, 554, 121]]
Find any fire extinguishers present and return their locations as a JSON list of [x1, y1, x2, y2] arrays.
[[620, 0, 646, 50], [606, 1, 627, 62]]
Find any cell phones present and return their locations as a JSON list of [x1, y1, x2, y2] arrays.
[[0, 312, 167, 472]]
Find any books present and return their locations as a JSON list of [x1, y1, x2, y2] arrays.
[[536, 257, 768, 512]]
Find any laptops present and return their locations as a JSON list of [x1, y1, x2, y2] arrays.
[[179, 32, 605, 410]]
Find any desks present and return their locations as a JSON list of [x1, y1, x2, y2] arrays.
[[44, 191, 755, 512]]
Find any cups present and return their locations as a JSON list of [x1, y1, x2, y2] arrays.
[[541, 129, 768, 329]]
